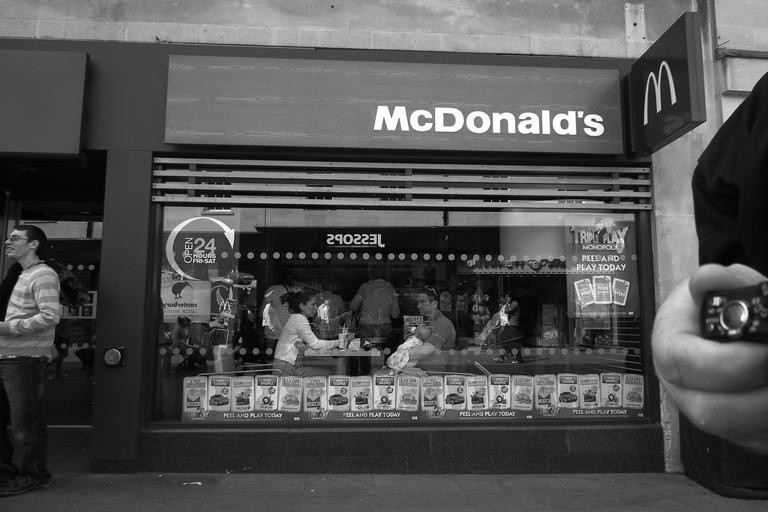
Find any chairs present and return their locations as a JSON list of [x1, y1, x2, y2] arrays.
[[197, 344, 283, 376]]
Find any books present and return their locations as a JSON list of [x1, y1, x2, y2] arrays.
[[181, 372, 643, 413]]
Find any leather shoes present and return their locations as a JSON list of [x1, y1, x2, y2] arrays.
[[0, 469, 54, 496]]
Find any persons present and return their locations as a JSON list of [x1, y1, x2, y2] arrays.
[[648, 69, 766, 458], [493, 293, 513, 360], [308, 278, 345, 341], [173, 315, 198, 372], [385, 324, 431, 373], [490, 292, 524, 364], [272, 290, 354, 377], [349, 265, 400, 338], [257, 270, 305, 375], [371, 285, 456, 377], [0, 222, 63, 496]]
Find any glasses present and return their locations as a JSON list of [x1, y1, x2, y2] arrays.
[[4, 236, 30, 242], [424, 284, 439, 297]]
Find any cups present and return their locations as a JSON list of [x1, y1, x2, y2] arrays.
[[338, 333, 349, 351]]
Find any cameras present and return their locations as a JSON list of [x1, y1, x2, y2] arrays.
[[702, 280, 767, 346]]
